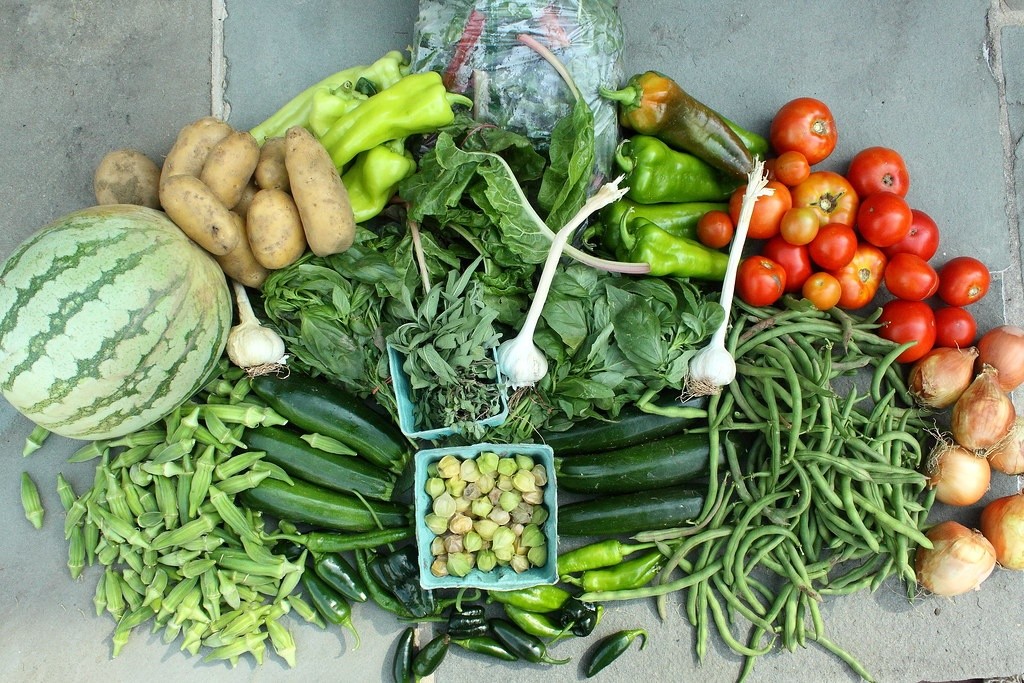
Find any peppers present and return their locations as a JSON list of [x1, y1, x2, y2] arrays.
[[246, 50, 474, 225], [257, 521, 690, 683], [599, 71, 769, 281]]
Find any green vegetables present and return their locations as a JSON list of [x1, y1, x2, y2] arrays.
[[393, 0, 648, 275], [260, 224, 726, 445]]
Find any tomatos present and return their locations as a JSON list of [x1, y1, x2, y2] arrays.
[[698, 96, 991, 363]]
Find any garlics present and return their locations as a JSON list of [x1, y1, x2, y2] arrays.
[[495, 338, 548, 388], [225, 280, 285, 378], [687, 343, 736, 396]]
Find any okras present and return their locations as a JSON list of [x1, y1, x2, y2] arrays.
[[20, 360, 359, 668]]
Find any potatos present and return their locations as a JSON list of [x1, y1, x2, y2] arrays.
[[95, 117, 356, 288]]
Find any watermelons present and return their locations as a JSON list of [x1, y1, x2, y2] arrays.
[[0, 204, 233, 441]]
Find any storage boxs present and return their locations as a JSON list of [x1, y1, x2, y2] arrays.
[[414, 442, 560, 591], [385, 340, 509, 439]]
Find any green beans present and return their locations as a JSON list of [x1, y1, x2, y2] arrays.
[[579, 306, 937, 683]]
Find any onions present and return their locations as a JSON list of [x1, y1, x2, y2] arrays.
[[906, 324, 1024, 596]]
